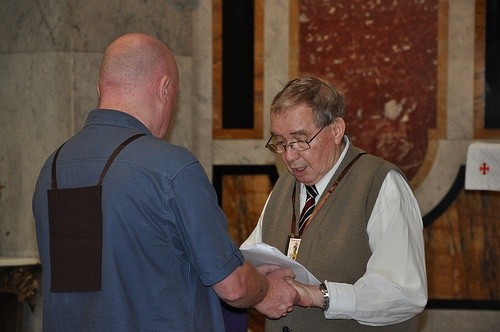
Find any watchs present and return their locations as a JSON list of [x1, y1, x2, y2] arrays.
[[318, 282, 332, 317]]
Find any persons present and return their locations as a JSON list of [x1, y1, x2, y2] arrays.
[[239, 77, 429, 327], [32, 30, 302, 330]]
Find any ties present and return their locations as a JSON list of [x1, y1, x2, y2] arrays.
[[298, 184, 318, 238]]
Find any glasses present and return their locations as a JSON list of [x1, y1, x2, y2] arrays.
[[265, 119, 334, 154]]
